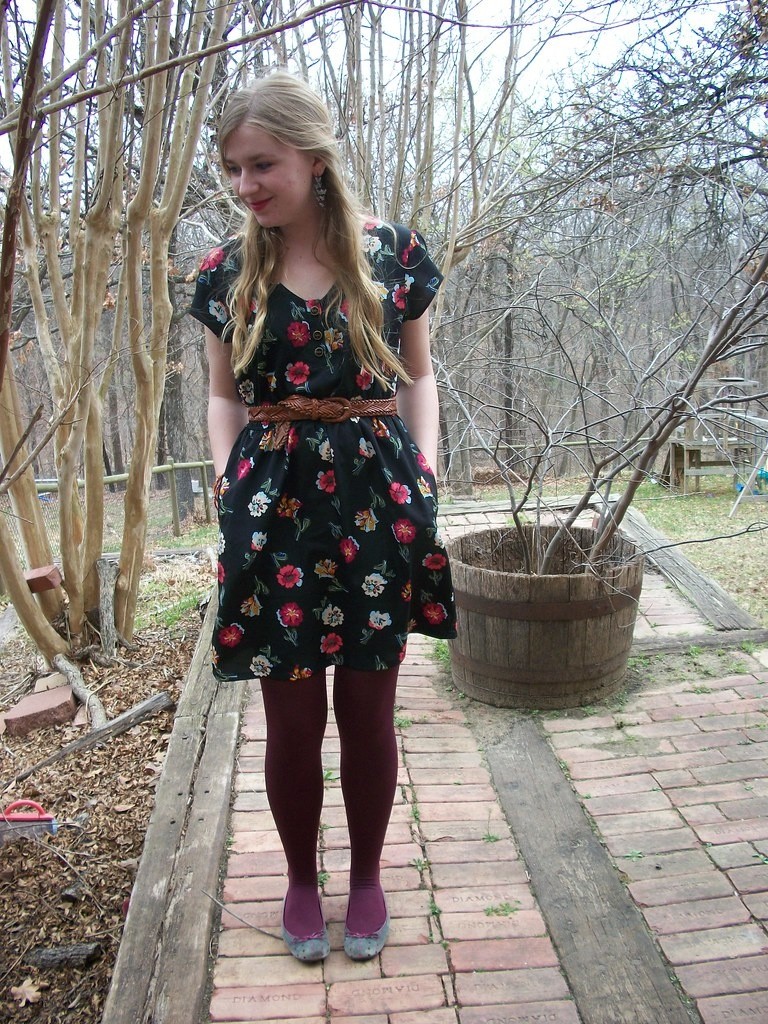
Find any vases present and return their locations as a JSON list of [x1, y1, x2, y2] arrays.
[[443, 524, 644, 709]]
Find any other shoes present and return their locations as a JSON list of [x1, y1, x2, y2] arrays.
[[282, 886, 330, 961], [344, 881, 390, 962]]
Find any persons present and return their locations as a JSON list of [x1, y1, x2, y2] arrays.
[[189, 73, 459, 961]]
